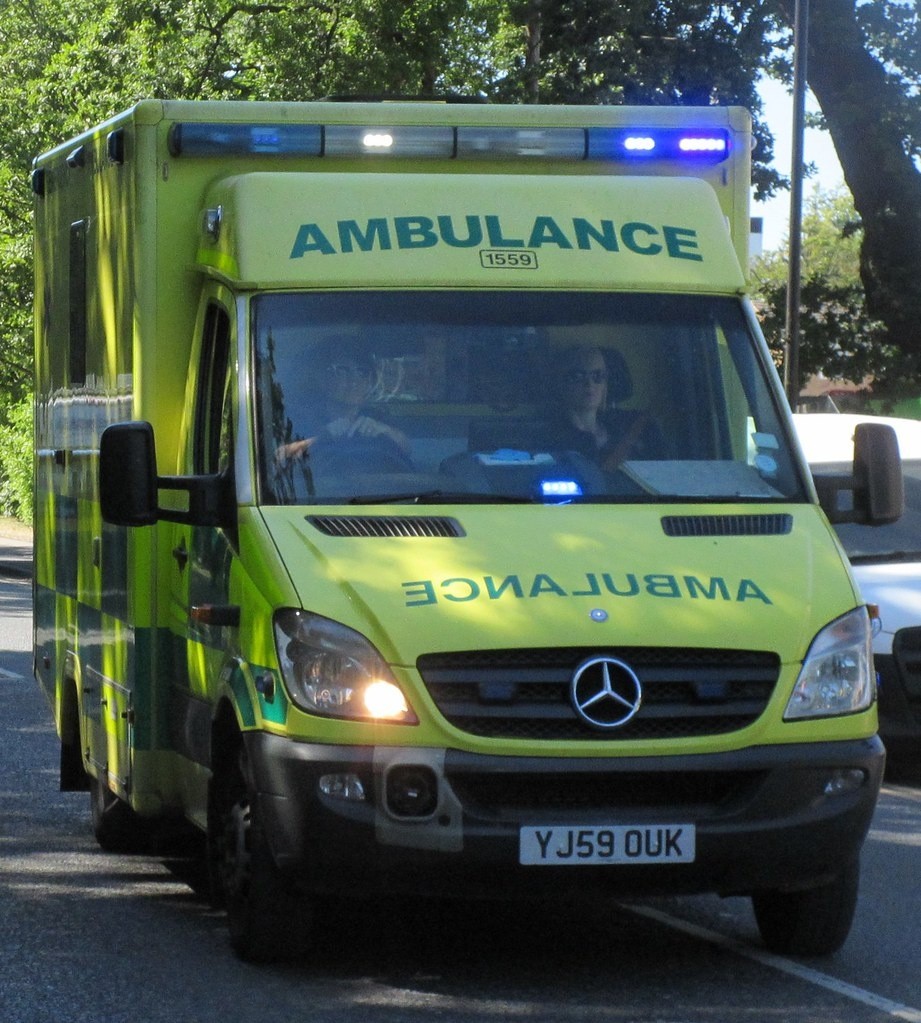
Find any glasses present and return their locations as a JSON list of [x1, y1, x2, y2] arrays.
[[329, 363, 372, 381], [562, 368, 609, 388]]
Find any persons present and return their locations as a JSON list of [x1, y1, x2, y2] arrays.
[[512, 335, 672, 477], [261, 329, 417, 487]]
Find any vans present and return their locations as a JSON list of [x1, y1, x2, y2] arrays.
[[741, 411, 921, 774]]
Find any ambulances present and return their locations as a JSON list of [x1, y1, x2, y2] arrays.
[[28, 98, 905, 963]]
